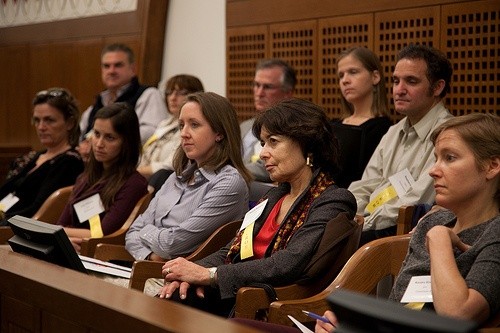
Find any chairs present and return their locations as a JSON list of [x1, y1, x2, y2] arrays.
[[0, 176, 416, 325]]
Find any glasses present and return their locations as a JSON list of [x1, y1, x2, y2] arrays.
[[36, 91, 65, 97]]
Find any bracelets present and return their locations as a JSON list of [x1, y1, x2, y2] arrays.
[[210, 267, 217, 285]]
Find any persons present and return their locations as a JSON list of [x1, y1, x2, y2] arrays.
[[154, 97, 357, 317], [87, 91, 250, 297], [76, 42, 166, 159], [0, 88, 85, 226], [347, 45, 453, 247], [315, 113, 500, 333], [240, 59, 298, 181], [59, 103, 149, 252], [136, 73, 205, 179], [336, 47, 393, 188]]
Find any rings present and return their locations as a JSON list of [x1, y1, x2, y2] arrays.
[[166, 267, 169, 273]]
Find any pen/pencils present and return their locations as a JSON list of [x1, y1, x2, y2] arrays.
[[302, 310, 330, 323]]
